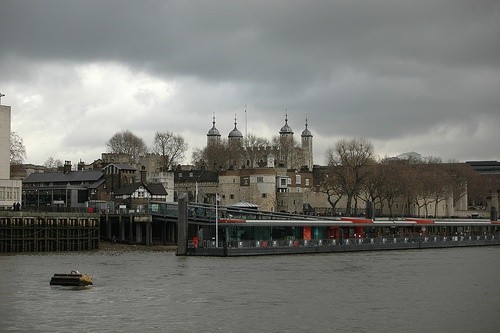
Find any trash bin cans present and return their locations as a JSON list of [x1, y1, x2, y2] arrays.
[[83, 206, 87, 213], [490, 206, 497, 221], [472, 213, 479, 218]]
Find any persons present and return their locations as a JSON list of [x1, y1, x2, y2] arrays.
[[291, 211, 374, 217]]
